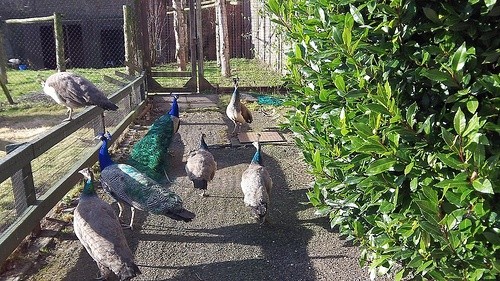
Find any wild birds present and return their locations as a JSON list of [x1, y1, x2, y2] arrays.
[[40, 71, 119, 122], [225, 76, 253, 136], [124, 93, 180, 185], [93, 131, 196, 232], [240, 134, 273, 227], [185, 132, 218, 197], [73, 167, 142, 281]]
